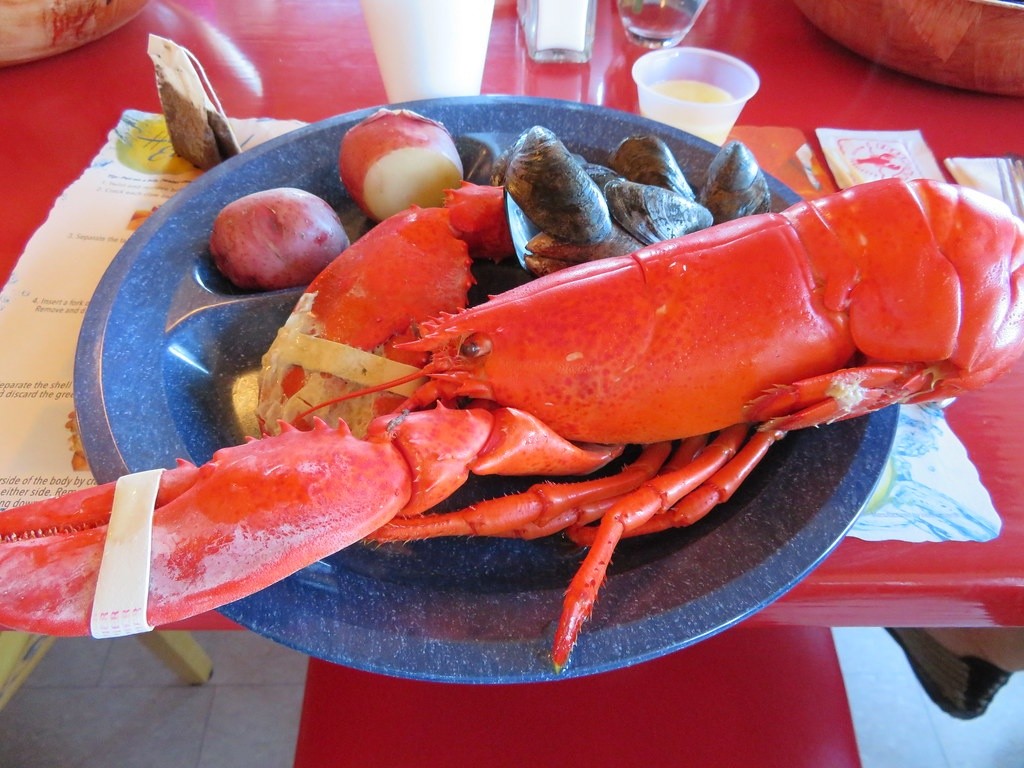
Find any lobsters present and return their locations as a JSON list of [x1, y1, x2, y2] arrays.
[[0, 179, 1024, 674]]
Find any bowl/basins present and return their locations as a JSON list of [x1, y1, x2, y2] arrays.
[[0, 1, 149, 65], [795, 1, 1024, 99]]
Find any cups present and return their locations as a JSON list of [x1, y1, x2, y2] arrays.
[[614, 0, 708, 50], [630, 46, 762, 148], [359, 0, 496, 105]]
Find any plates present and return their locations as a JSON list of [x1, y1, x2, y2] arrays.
[[71, 95, 898, 684]]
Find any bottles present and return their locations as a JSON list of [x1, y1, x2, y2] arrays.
[[515, 0, 597, 63]]
